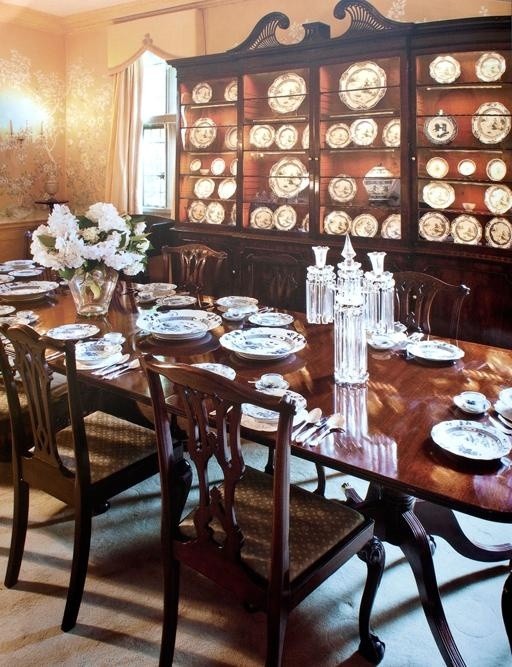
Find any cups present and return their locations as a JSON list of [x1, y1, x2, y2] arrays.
[[461, 203, 475, 214], [261, 373, 284, 388], [16, 309, 36, 320], [104, 332, 123, 343]]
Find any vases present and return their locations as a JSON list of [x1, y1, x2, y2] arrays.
[[67, 265, 119, 319], [45, 171, 59, 197]]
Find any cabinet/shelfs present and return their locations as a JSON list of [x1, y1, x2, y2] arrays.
[[165, 0, 512, 346]]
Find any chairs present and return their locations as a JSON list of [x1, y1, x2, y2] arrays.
[[137, 351, 387, 667], [0, 321, 195, 632], [161, 242, 228, 297], [387, 272, 471, 339], [0, 356, 82, 465]]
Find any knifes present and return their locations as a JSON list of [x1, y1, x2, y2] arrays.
[[295, 414, 333, 443]]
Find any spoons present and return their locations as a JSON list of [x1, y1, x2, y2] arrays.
[[287, 407, 322, 442], [93, 353, 140, 380], [310, 414, 345, 446]]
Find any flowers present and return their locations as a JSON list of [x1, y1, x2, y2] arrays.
[[30, 201, 155, 304]]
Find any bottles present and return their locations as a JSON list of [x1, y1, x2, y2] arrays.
[[305, 235, 407, 385], [362, 162, 395, 203]]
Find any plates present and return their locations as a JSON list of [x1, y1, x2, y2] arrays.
[[324, 118, 380, 148], [430, 386, 511, 461], [423, 111, 459, 145], [326, 173, 359, 205], [187, 77, 237, 225], [428, 55, 462, 83], [406, 341, 465, 362], [458, 159, 477, 176], [324, 209, 402, 239], [336, 61, 389, 110], [265, 73, 307, 114], [483, 159, 510, 248], [250, 124, 298, 149], [190, 363, 236, 380], [28, 315, 41, 324], [473, 52, 507, 82], [74, 337, 127, 366], [418, 156, 455, 242], [248, 206, 298, 231], [451, 214, 482, 245], [0, 296, 61, 312], [240, 380, 307, 421], [470, 101, 511, 145], [267, 154, 308, 201], [0, 317, 30, 326], [133, 283, 307, 361], [301, 122, 309, 148], [301, 214, 309, 233], [0, 260, 61, 301], [46, 324, 101, 340], [0, 305, 16, 316], [381, 120, 401, 147]]
